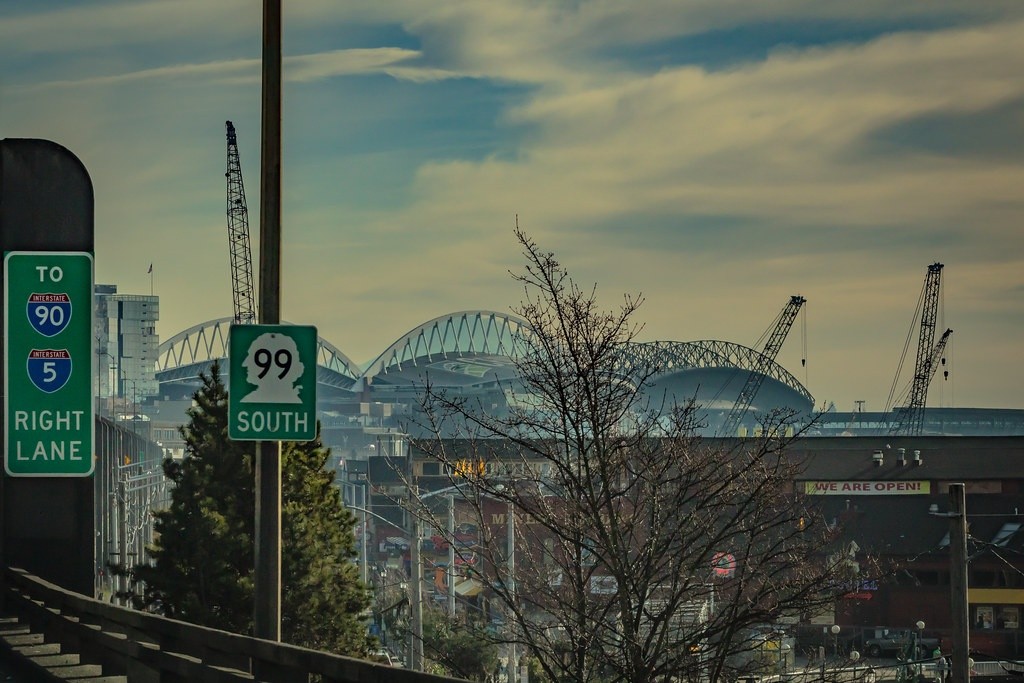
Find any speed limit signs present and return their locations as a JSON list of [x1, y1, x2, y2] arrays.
[[26, 291, 74, 338], [27, 347, 74, 393]]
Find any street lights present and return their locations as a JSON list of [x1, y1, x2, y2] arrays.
[[780, 643, 792, 674], [850, 650, 861, 683], [776, 629, 784, 660], [916, 620, 926, 663], [830, 624, 841, 664]]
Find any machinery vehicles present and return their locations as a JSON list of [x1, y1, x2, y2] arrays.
[[219, 116, 256, 323], [695, 293, 813, 436], [868, 262, 956, 432]]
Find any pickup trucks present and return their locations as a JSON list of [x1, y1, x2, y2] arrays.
[[866, 629, 939, 661]]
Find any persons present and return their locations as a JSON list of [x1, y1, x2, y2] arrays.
[[807, 646, 816, 668], [907, 646, 977, 678]]
